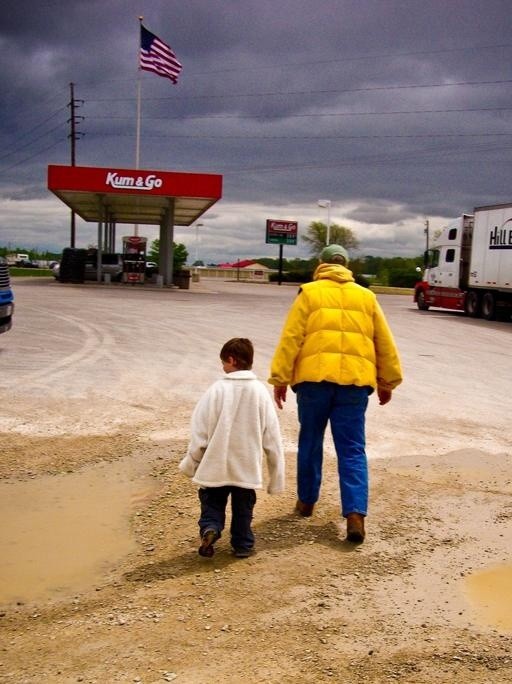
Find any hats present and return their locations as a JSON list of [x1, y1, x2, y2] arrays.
[[320, 244, 349, 268]]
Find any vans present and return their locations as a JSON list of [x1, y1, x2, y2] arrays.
[[49, 253, 123, 280]]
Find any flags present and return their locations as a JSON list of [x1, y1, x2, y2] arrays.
[[138, 23, 182, 86]]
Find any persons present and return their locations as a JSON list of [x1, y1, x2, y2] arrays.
[[179, 337, 286, 558], [268, 244, 404, 546]]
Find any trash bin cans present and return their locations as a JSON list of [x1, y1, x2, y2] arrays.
[[174, 270, 190, 289]]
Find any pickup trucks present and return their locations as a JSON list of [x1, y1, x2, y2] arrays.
[[16, 259, 32, 268]]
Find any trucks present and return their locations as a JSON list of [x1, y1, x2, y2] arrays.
[[414, 202, 512, 320]]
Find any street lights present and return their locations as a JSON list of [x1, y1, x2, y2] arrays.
[[318, 197, 331, 245], [195, 223, 203, 271]]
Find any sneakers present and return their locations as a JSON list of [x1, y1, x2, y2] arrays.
[[199, 526, 218, 557], [234, 547, 250, 558], [347, 513, 365, 543], [296, 498, 314, 517]]
